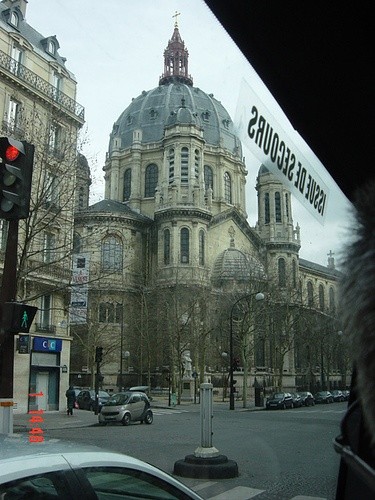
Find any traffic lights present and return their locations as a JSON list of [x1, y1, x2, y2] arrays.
[[96, 346, 104, 362], [0, 136, 35, 217], [6, 302, 39, 333], [99, 375, 104, 382]]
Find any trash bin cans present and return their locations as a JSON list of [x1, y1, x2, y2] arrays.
[[255, 387, 264, 406]]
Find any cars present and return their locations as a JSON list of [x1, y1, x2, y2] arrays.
[[71, 386, 154, 426], [0, 433, 202, 500], [266, 390, 350, 410]]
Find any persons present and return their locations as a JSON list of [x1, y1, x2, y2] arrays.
[[65, 386, 76, 415]]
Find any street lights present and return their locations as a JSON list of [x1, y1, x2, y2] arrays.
[[321, 331, 343, 392], [222, 351, 228, 401], [230, 290, 264, 410], [124, 350, 129, 391]]
